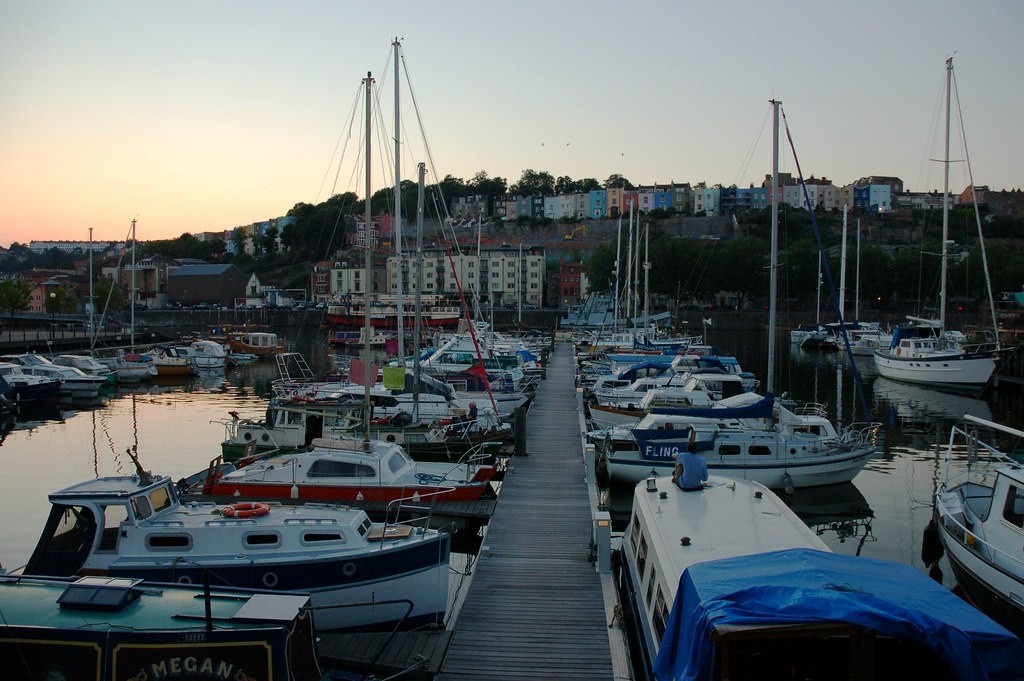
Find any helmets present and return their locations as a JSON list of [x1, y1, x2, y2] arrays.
[[469, 403, 475, 409]]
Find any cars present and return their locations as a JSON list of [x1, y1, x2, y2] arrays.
[[703, 303, 720, 311], [297, 302, 308, 311], [680, 303, 698, 311], [652, 303, 667, 312], [315, 302, 325, 310]]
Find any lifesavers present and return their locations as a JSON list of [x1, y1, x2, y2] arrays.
[[294, 395, 315, 403], [371, 419, 389, 424], [222, 503, 270, 517]]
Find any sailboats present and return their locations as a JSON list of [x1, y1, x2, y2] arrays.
[[0, 31, 1023, 680]]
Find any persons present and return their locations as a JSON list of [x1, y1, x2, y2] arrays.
[[513, 299, 518, 312], [672, 443, 708, 489], [466, 402, 478, 428]]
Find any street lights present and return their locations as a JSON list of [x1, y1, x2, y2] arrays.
[[49, 292, 57, 338]]
